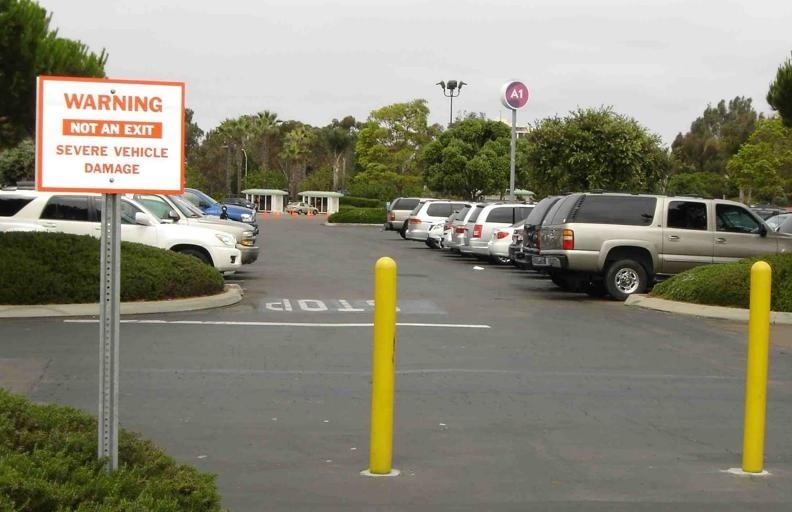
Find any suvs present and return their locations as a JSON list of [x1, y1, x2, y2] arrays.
[[384, 197, 536, 264], [523, 191, 792, 300]]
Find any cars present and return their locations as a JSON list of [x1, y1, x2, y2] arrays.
[[0, 185, 257, 275], [286, 202, 318, 215]]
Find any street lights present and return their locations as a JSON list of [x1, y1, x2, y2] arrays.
[[436, 80, 466, 127]]
